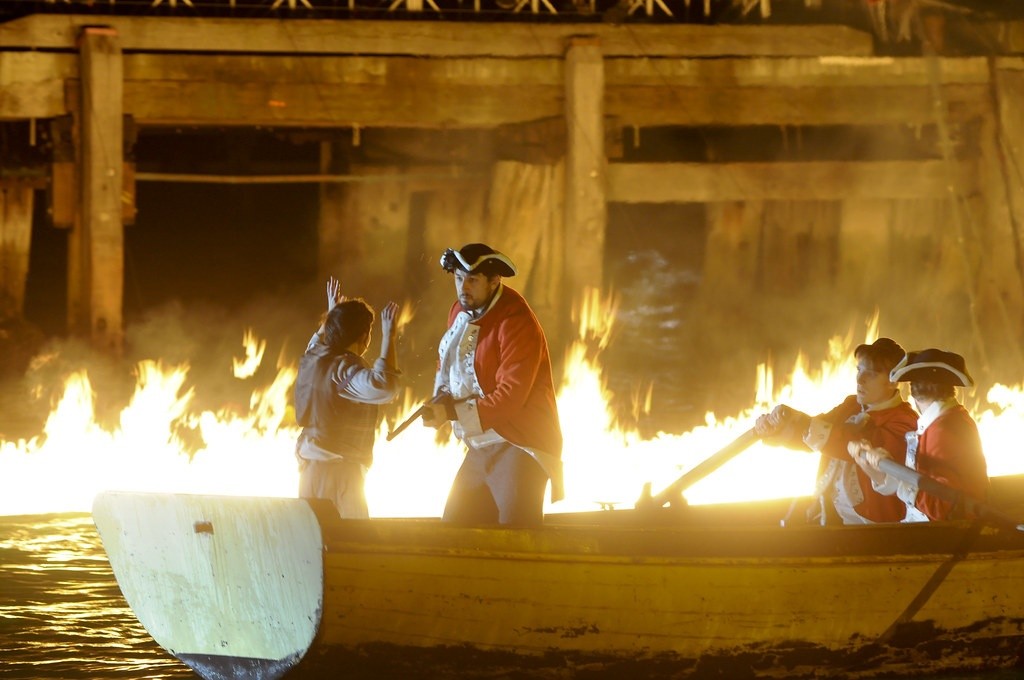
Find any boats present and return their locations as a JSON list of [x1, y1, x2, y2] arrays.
[[89, 489, 1024, 678]]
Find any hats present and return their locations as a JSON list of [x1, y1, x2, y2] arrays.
[[439, 243, 518, 277], [889, 348, 974, 387]]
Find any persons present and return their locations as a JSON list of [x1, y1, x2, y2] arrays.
[[385, 240, 568, 529], [753, 337, 995, 521], [293, 276, 403, 521]]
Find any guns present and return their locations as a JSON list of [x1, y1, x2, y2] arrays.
[[385, 384, 458, 441]]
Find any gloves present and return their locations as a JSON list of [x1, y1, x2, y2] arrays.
[[847, 437, 874, 459], [865, 447, 895, 468], [766, 403, 786, 426], [754, 413, 770, 434]]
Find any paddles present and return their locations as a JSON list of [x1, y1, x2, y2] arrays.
[[634, 410, 791, 509], [858, 450, 1024, 536]]
